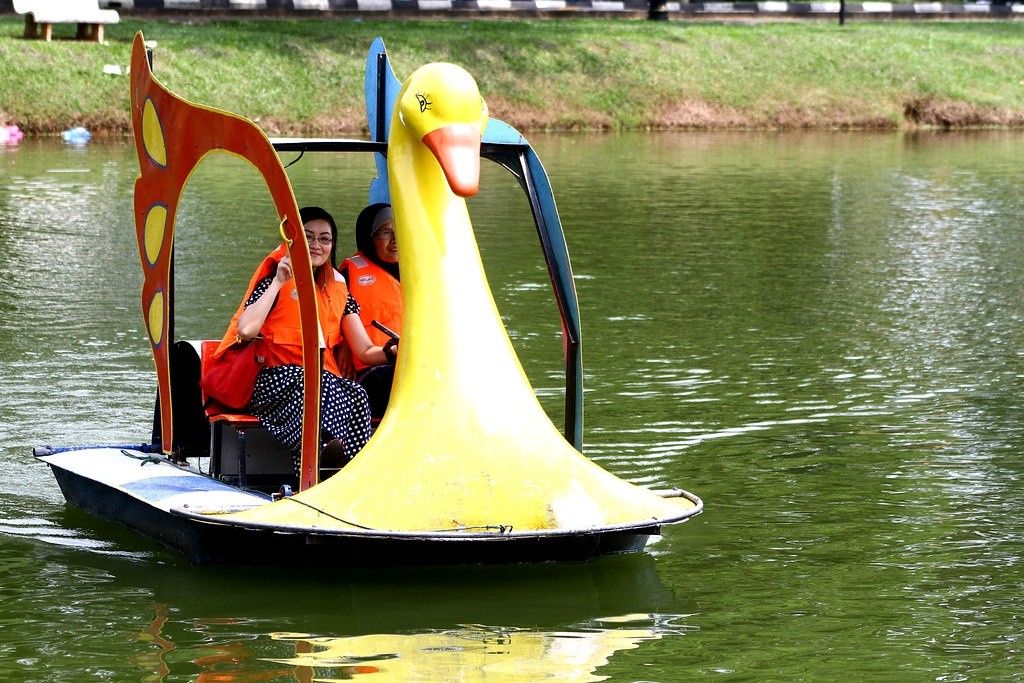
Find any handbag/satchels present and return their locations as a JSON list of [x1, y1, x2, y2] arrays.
[[199, 342, 266, 409]]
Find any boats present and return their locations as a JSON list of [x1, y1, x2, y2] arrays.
[[29, 29, 703, 548]]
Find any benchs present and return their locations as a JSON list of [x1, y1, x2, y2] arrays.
[[11, 0, 120, 44], [200, 340, 384, 495]]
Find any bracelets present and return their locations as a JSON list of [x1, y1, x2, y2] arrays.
[[383, 338, 397, 364]]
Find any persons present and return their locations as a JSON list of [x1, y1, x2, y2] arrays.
[[337, 203, 400, 428], [213, 206, 400, 484]]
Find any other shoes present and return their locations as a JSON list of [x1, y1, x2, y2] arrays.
[[319, 439, 344, 481]]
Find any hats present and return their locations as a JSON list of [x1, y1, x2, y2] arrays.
[[371, 207, 393, 236]]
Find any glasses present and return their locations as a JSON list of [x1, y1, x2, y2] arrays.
[[372, 228, 395, 240], [305, 231, 335, 245]]
